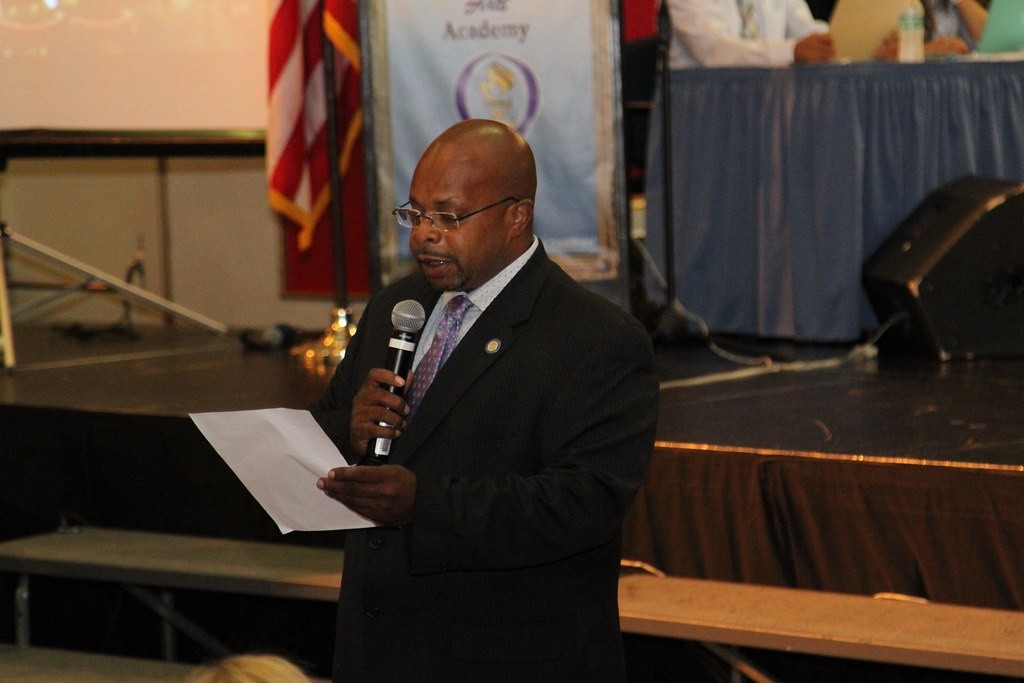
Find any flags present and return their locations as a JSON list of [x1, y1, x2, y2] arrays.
[[266, 0, 364, 250]]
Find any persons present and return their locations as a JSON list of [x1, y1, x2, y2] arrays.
[[874, 0, 989, 61], [666, 0, 839, 71], [307, 119, 660, 683]]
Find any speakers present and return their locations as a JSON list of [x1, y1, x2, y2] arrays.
[[863, 172, 1024, 359]]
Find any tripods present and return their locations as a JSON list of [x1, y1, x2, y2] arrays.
[[649, 0, 795, 364]]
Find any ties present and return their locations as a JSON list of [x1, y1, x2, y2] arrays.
[[395, 295, 475, 431]]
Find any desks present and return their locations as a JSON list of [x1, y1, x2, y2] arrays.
[[644, 59, 1022, 342], [1, 525, 1024, 681]]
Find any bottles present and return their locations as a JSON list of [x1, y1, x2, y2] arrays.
[[899, 0, 925, 63]]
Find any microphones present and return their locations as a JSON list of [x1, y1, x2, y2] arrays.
[[365, 298, 426, 465]]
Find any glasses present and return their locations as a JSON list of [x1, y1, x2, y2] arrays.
[[388, 195, 519, 234]]
